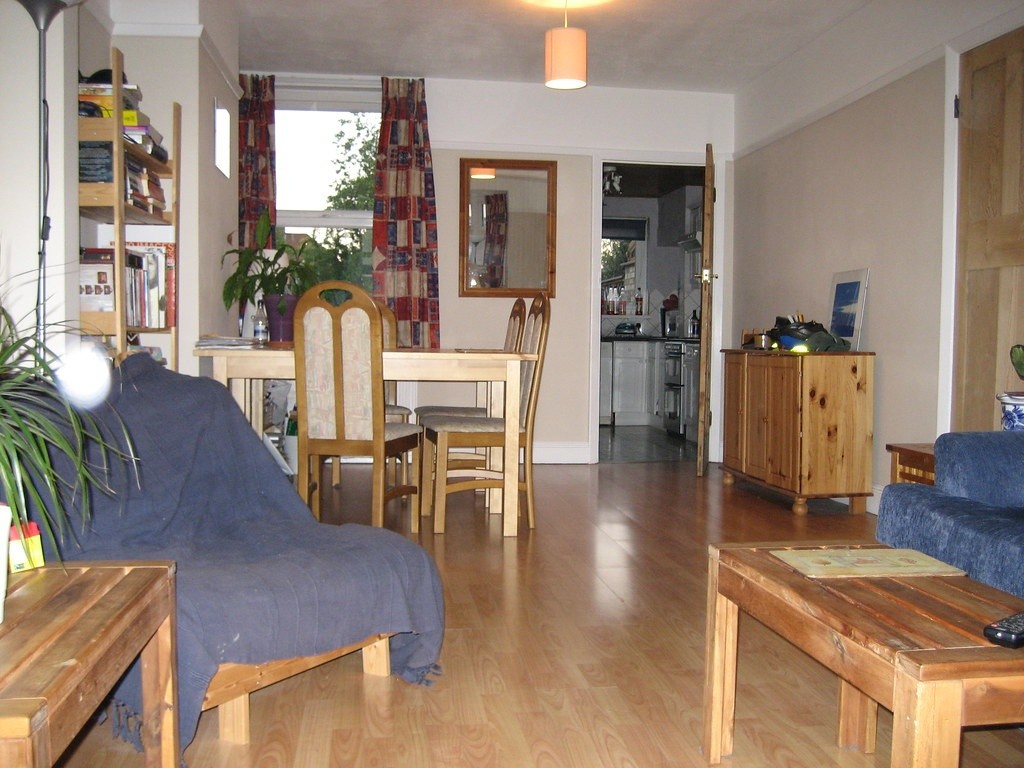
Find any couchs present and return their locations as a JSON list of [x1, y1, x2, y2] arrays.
[[871, 431, 1023, 595]]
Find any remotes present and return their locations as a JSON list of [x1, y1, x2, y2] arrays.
[[983, 612, 1024, 650]]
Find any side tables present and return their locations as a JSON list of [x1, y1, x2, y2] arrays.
[[0, 557, 183, 768], [885, 436, 943, 496]]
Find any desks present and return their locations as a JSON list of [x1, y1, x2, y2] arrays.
[[197, 347, 549, 536]]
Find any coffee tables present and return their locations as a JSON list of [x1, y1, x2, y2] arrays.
[[701, 536, 1024, 767]]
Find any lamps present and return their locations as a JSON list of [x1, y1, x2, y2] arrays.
[[602, 166, 622, 205], [470, 166, 497, 180], [543, 0, 589, 90]]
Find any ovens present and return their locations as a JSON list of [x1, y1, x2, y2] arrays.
[[664, 343, 685, 436]]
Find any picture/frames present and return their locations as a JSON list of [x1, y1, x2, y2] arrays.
[[827, 266, 872, 352]]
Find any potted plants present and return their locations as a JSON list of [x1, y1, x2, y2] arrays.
[[0, 247, 141, 625], [997, 345, 1024, 430], [217, 211, 364, 339]]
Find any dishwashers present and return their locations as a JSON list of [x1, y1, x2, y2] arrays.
[[684, 344, 700, 443]]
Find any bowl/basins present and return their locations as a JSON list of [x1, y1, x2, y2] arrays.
[[754, 334, 771, 351]]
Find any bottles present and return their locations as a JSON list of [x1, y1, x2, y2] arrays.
[[690, 310, 700, 338], [254, 300, 268, 349], [607, 286, 626, 314], [634, 287, 643, 315]]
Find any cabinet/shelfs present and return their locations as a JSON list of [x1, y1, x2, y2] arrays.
[[684, 344, 698, 442], [79, 45, 188, 370], [718, 349, 878, 513], [601, 341, 664, 429]]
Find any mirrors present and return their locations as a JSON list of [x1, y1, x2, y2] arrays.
[[457, 158, 558, 298]]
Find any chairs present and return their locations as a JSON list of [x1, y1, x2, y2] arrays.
[[421, 299, 552, 533], [9, 358, 445, 752], [294, 281, 420, 531], [414, 300, 526, 514], [311, 299, 405, 489]]
